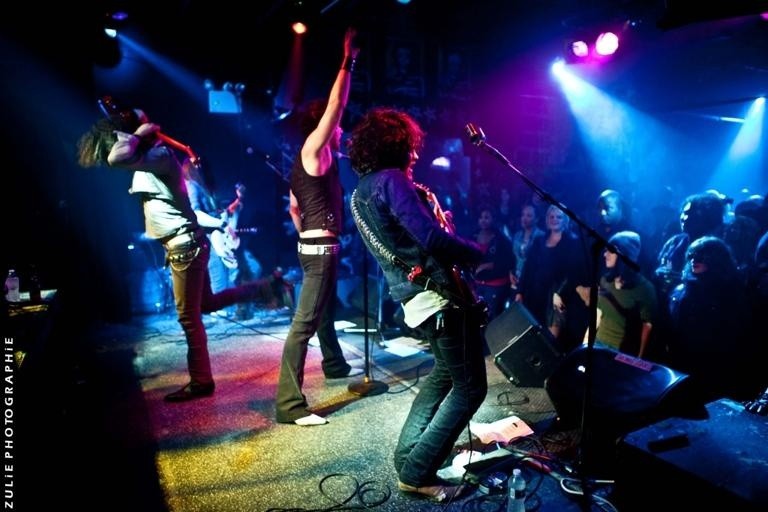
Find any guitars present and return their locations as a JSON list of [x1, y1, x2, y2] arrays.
[[98, 94, 201, 169], [211, 184, 244, 268], [413, 182, 489, 327]]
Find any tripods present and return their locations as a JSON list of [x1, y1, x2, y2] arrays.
[[480, 141, 641, 512], [341, 273, 403, 348]]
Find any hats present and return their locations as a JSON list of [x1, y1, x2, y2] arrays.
[[604, 229, 642, 265]]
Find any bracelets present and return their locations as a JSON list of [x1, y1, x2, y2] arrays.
[[338, 53, 357, 75]]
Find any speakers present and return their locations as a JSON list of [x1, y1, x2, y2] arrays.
[[347, 277, 405, 328], [614, 397, 768, 511], [485, 300, 567, 387], [544, 339, 703, 444]]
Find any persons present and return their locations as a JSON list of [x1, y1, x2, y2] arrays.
[[276, 25, 361, 427], [182, 140, 768, 419], [77, 100, 215, 403], [346, 109, 497, 501]]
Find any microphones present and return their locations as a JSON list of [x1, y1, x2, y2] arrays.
[[464, 122, 487, 146]]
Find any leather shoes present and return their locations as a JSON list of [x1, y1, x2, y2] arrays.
[[293, 412, 329, 426], [396, 474, 466, 503], [347, 367, 365, 377], [163, 379, 216, 403], [269, 264, 297, 311]]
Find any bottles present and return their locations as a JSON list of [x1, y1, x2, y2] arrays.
[[507, 468, 527, 512], [6, 269, 21, 302]]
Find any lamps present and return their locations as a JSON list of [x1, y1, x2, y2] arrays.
[[562, 16, 638, 67]]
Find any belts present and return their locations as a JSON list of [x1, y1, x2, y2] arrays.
[[297, 241, 340, 255], [168, 247, 206, 263]]
